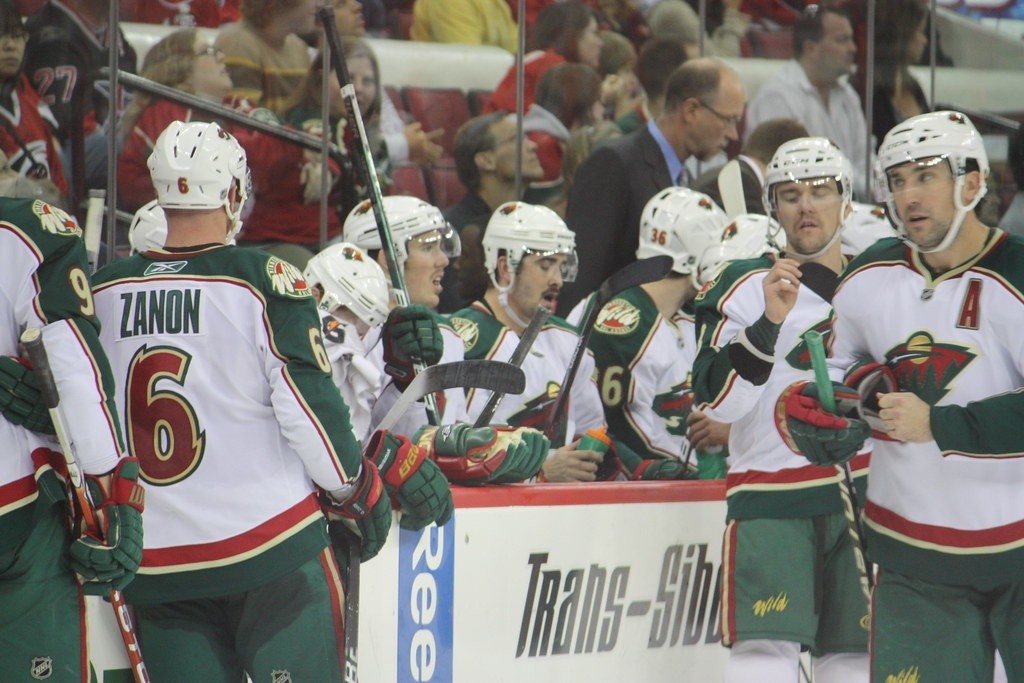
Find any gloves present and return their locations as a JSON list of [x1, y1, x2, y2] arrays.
[[0, 355, 56, 435], [843, 357, 900, 441], [774, 380, 871, 468], [365, 429, 455, 531], [319, 458, 392, 563], [633, 460, 697, 480], [417, 423, 551, 486], [68, 457, 146, 596]]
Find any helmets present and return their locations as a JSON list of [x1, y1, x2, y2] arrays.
[[128, 199, 168, 256], [343, 195, 446, 277], [878, 111, 990, 226], [147, 121, 247, 220], [694, 213, 787, 290], [635, 186, 730, 274], [302, 242, 390, 328], [481, 201, 576, 291], [760, 137, 854, 225]]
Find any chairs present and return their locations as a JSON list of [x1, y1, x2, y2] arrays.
[[384, 86, 496, 207], [739, 30, 794, 59]]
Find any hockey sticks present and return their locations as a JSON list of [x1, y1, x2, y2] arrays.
[[340, 358, 525, 680], [544, 253, 674, 438], [22, 324, 151, 683], [470, 303, 553, 428], [802, 330, 876, 605], [320, 1, 443, 435]]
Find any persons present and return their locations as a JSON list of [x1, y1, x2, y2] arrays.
[[0, 0, 930, 322], [0, 196, 144, 683], [774, 110, 1024, 683], [565, 186, 899, 481], [85, 120, 392, 683], [302, 195, 609, 531], [997, 122, 1024, 236], [691, 137, 899, 683]]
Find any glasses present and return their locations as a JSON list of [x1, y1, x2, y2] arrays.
[[700, 101, 742, 127], [17, 31, 31, 44], [193, 46, 226, 63]]
